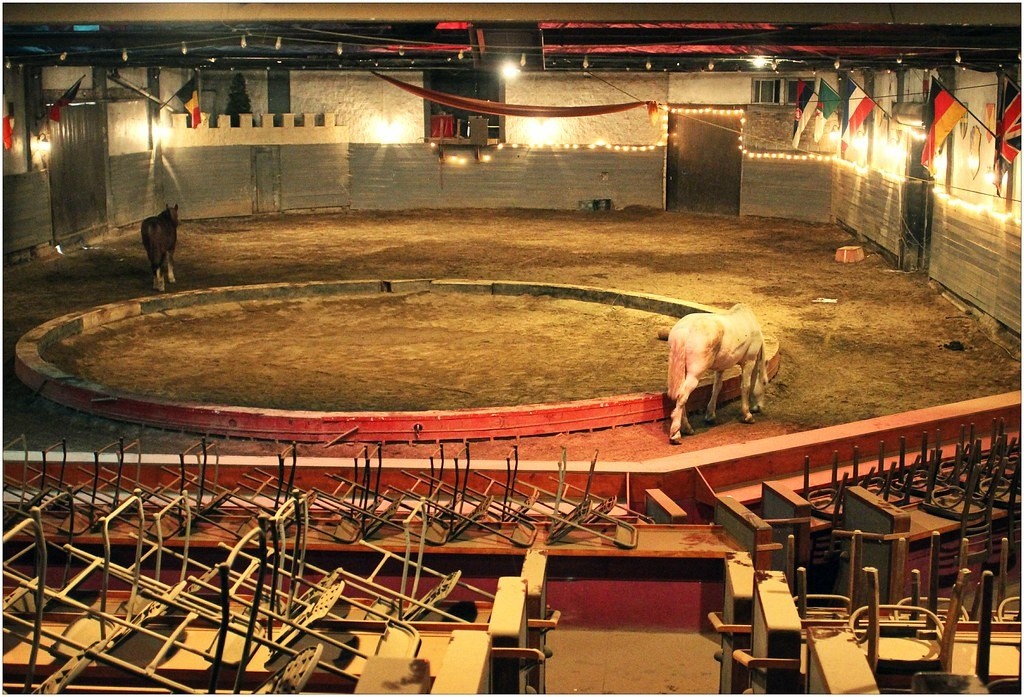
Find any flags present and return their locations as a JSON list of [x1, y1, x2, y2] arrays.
[[3, 84, 16, 151], [47, 77, 83, 122], [174, 72, 202, 129], [992, 71, 1021, 199], [791, 79, 819, 149], [920, 78, 968, 178], [840, 78, 876, 154], [813, 79, 843, 143]]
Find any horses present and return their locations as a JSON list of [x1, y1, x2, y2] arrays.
[[140, 204, 179, 293], [667, 303, 769, 445]]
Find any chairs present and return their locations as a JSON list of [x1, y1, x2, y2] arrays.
[[3, 433, 642, 694], [705, 414, 1021, 695]]
